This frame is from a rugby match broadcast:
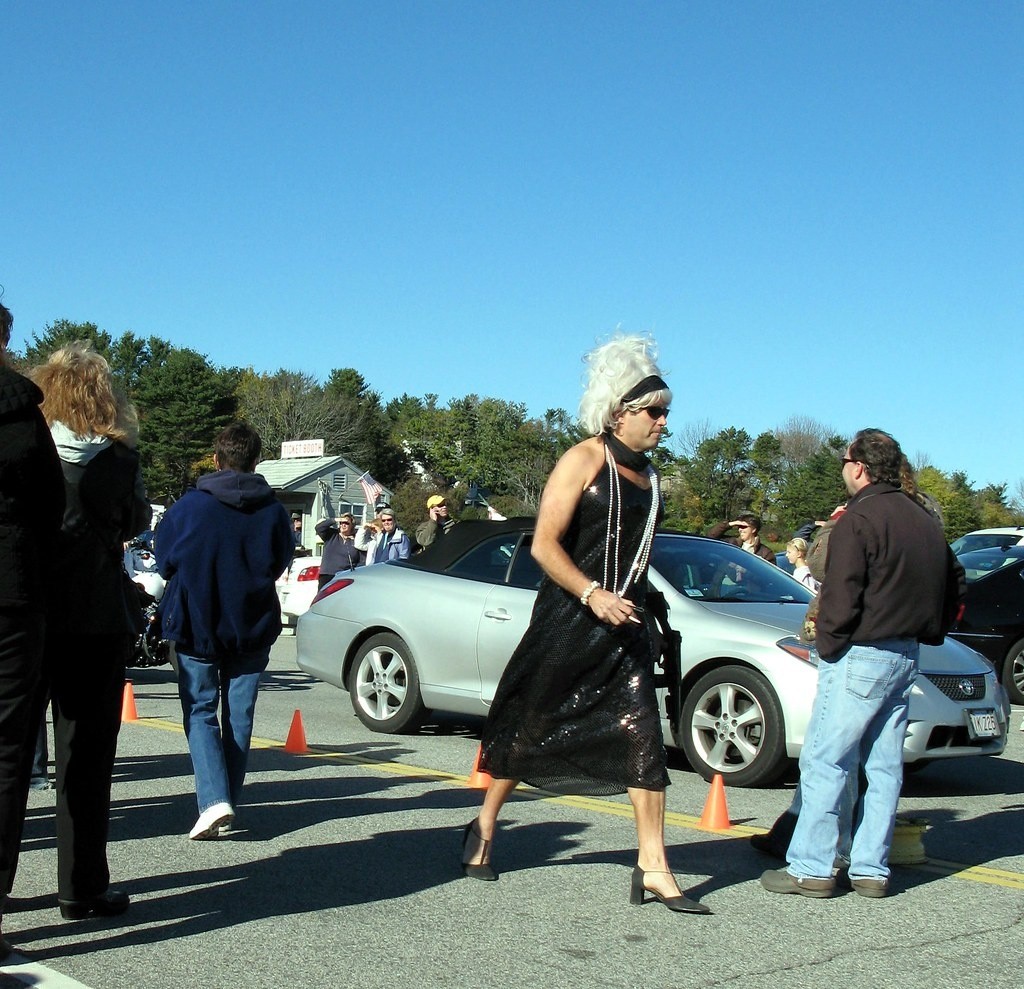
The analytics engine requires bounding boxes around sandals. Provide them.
[760,869,837,898]
[839,867,888,898]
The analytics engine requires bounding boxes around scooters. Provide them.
[129,529,167,665]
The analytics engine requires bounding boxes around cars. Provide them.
[295,516,1012,788]
[278,556,323,618]
[947,527,1024,706]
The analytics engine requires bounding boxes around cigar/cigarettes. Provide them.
[628,616,641,624]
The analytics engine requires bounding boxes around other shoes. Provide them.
[219,824,232,832]
[29,779,55,790]
[189,802,235,840]
[751,834,785,860]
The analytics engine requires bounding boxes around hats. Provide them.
[374,502,391,513]
[427,495,447,508]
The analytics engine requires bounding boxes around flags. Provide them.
[360,473,382,504]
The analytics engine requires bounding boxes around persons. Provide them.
[0,303,68,963]
[354,502,411,566]
[416,495,460,552]
[751,428,965,898]
[154,423,296,839]
[708,514,776,598]
[784,537,821,592]
[6,347,153,920]
[290,510,301,545]
[315,513,360,592]
[460,338,710,912]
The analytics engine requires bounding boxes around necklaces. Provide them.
[603,444,659,598]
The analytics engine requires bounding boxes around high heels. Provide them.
[629,865,710,913]
[58,891,129,918]
[462,818,498,881]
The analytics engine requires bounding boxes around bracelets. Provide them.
[581,581,601,606]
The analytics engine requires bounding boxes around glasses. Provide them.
[382,519,393,522]
[631,406,669,420]
[842,457,869,469]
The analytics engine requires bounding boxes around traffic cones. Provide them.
[121,682,138,721]
[283,709,311,754]
[700,774,732,828]
